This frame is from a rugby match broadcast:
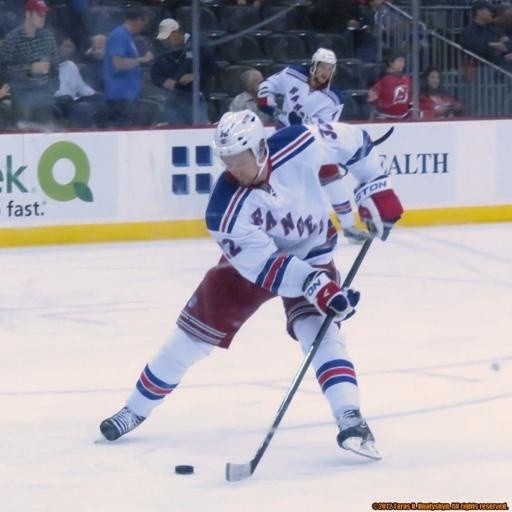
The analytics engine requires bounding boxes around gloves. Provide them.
[353,174,404,242]
[302,270,360,323]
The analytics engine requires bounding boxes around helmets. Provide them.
[310,47,337,75]
[213,110,266,158]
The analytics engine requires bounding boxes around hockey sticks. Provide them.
[226,230,375,480]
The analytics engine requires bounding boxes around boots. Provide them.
[100,405,150,441]
[335,406,375,449]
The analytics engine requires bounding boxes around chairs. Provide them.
[0,4,386,128]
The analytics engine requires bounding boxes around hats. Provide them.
[24,0,49,13]
[472,0,498,12]
[156,18,180,40]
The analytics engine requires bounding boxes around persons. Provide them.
[100,109,403,449]
[1,0,512,135]
[256,48,369,242]
